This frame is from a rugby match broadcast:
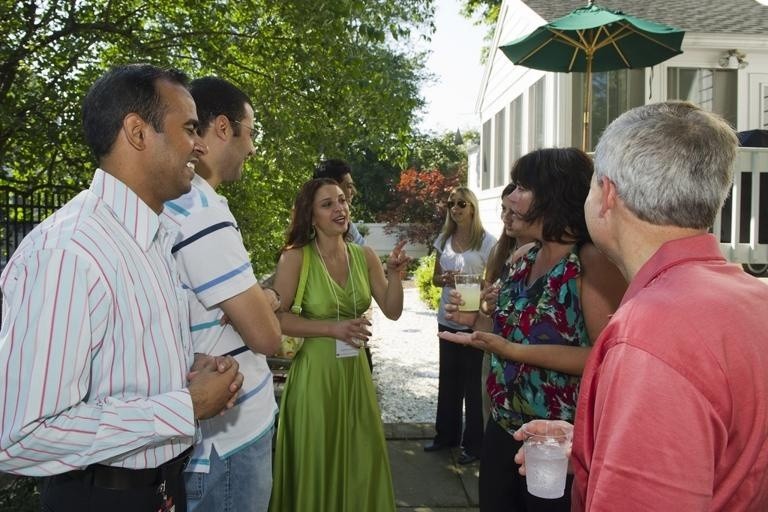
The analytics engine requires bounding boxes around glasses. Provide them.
[447,200,470,208]
[216,113,260,143]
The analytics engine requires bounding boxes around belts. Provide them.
[84,444,195,491]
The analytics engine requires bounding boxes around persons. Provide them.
[269,176,414,511]
[159,74,283,510]
[2,62,245,510]
[424,185,500,468]
[479,182,544,436]
[434,146,631,512]
[511,99,768,511]
[305,156,379,372]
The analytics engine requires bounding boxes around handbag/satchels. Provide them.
[266,245,311,360]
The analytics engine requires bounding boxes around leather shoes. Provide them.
[424,441,441,451]
[457,450,477,465]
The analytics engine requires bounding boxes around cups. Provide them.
[354,314,376,347]
[522,420,573,498]
[454,274,481,311]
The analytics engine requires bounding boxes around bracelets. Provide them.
[476,299,492,320]
[258,283,283,315]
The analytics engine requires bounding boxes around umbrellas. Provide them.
[497,1,688,153]
